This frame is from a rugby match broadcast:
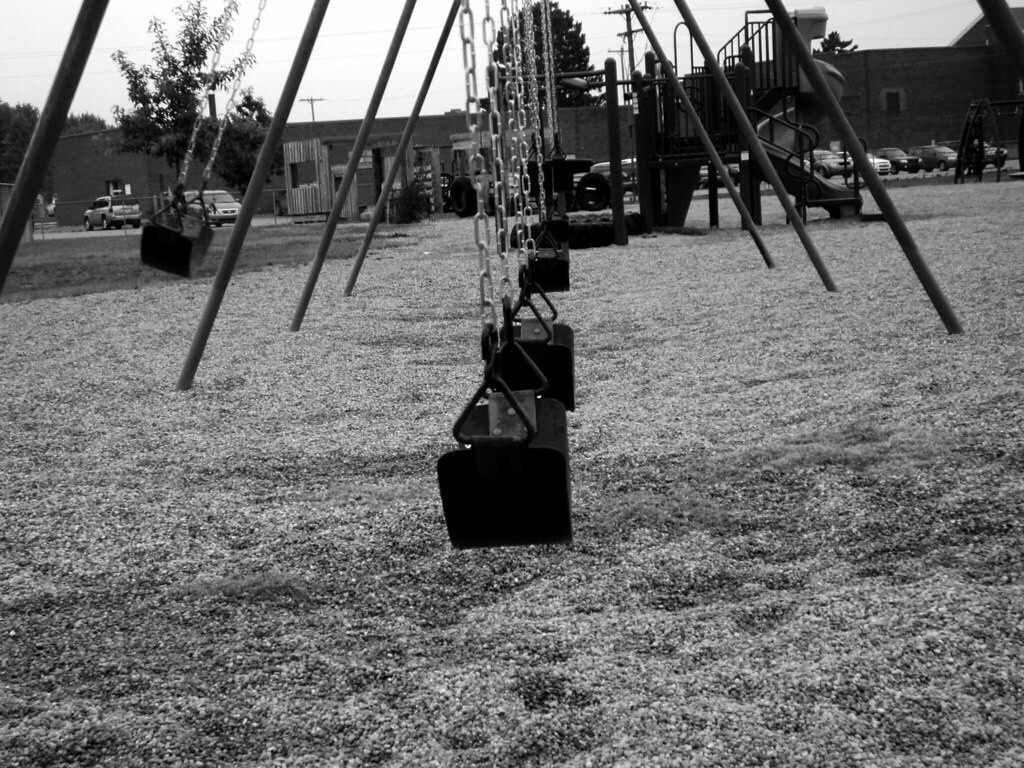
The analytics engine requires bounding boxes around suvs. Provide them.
[872,148,925,175]
[937,138,1008,168]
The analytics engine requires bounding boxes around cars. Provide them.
[82,194,141,230]
[804,150,856,180]
[837,151,892,175]
[180,189,242,228]
[566,154,744,199]
[46,196,56,216]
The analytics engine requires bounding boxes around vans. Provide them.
[907,145,959,172]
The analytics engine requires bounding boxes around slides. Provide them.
[748,55,863,213]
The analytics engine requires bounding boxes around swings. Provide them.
[431,0,580,554]
[136,0,268,280]
